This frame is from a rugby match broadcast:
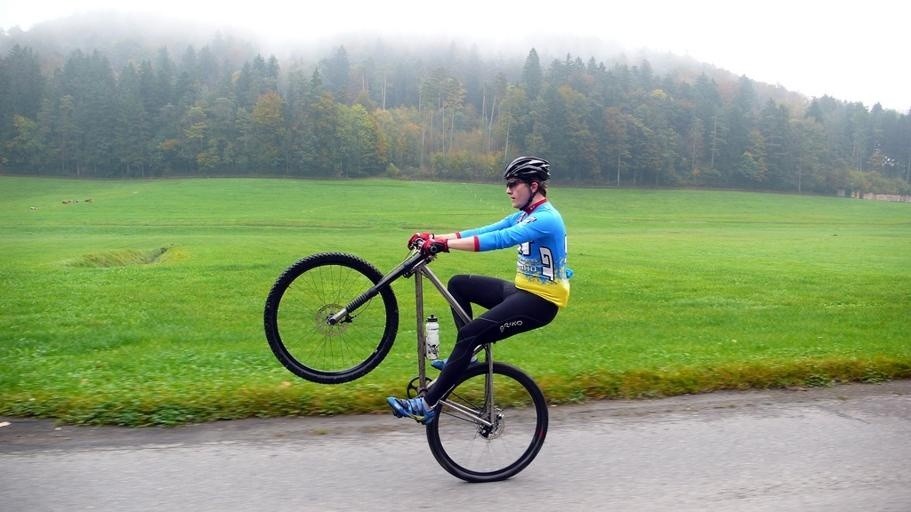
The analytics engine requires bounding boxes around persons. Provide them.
[385,154,574,427]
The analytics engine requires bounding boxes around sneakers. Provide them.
[386,393,438,427]
[431,354,480,372]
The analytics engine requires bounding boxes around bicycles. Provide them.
[262,231,550,484]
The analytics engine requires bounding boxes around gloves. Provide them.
[418,238,452,256]
[406,230,429,251]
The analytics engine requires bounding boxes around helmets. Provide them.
[503,154,552,184]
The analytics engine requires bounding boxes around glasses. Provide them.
[505,178,527,189]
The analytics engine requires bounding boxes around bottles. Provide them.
[425,314,441,360]
